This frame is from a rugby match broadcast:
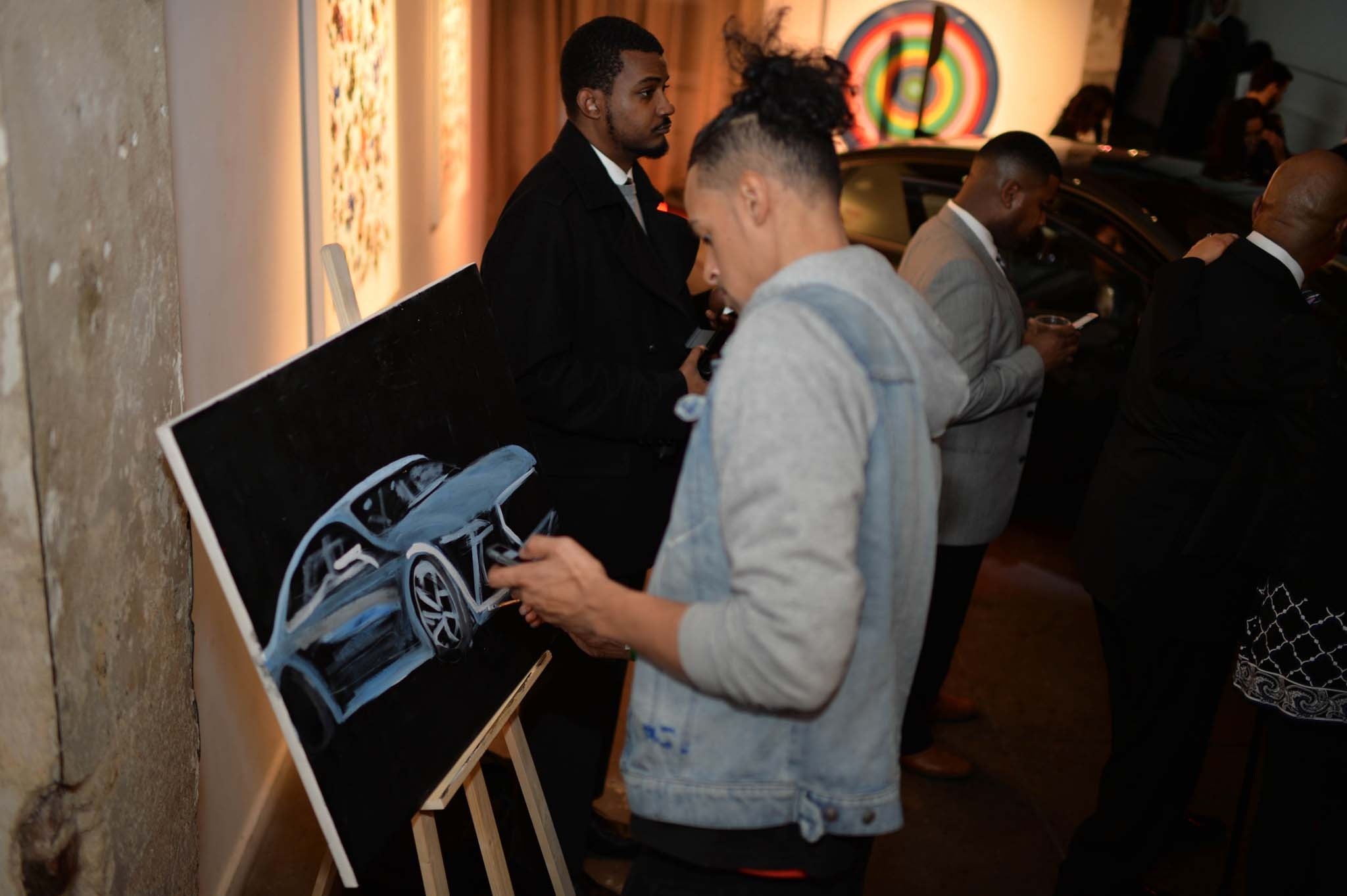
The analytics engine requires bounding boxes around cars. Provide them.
[262,444,562,760]
[837,132,1253,533]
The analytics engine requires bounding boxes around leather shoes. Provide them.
[931,690,979,719]
[900,744,973,780]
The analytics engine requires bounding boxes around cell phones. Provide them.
[1071,312,1099,330]
[485,542,536,567]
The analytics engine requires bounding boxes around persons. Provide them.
[901,131,1076,780]
[482,16,1347,896]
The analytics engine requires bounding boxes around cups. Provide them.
[1034,314,1072,339]
